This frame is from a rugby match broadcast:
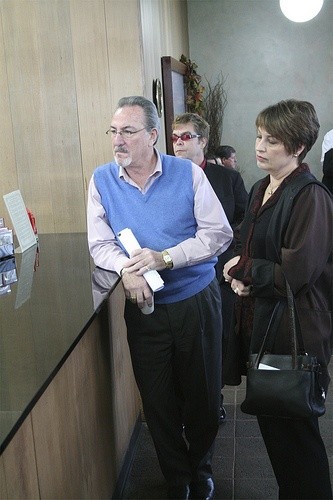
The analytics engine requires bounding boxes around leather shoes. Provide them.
[165,477,217,500]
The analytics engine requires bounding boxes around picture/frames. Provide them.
[162,55,188,156]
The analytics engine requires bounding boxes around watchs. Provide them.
[161,250,172,269]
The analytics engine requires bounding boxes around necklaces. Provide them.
[268,184,279,194]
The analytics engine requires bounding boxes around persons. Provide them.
[320,129,333,194]
[167,112,249,421]
[222,100,333,500]
[87,95,233,500]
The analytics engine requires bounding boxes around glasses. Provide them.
[169,131,200,142]
[105,126,147,137]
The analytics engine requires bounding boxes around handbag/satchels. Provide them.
[240,276,331,420]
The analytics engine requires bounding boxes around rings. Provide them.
[144,266,149,271]
[130,297,137,300]
[225,279,228,282]
[234,288,238,294]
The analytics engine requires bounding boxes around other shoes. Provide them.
[219,406,227,422]
